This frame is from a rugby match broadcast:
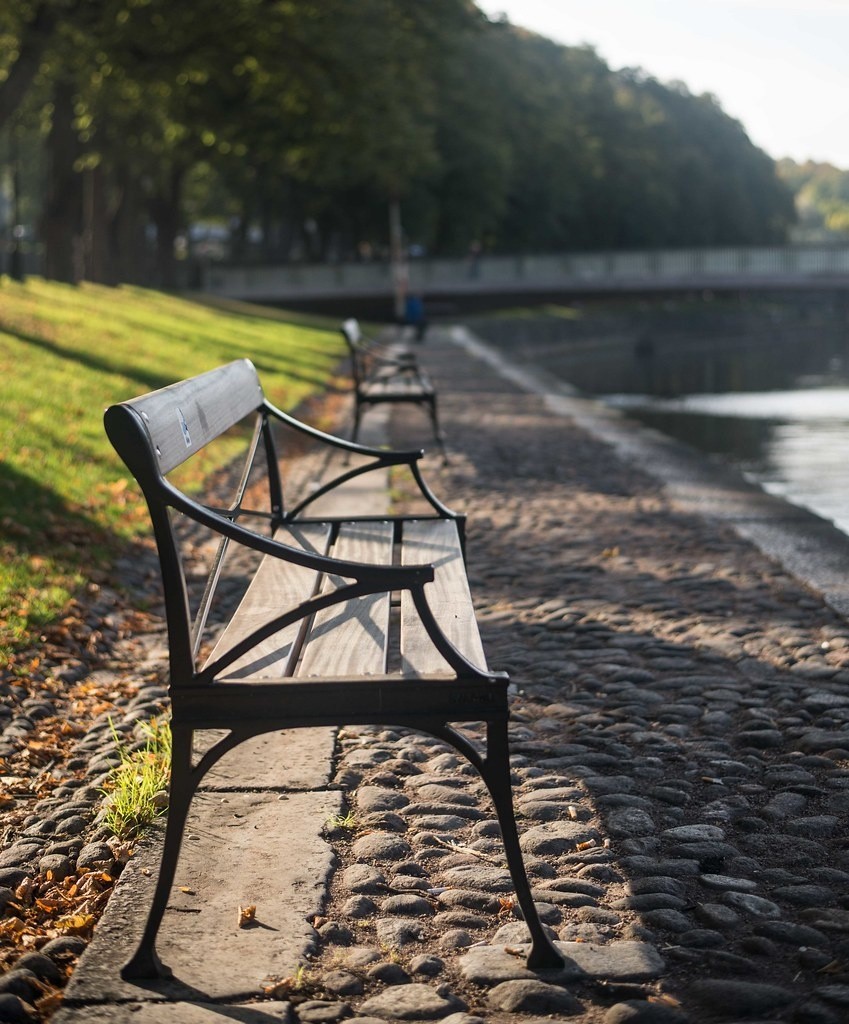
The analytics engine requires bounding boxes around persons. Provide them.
[405,292,426,342]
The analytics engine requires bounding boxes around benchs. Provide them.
[340,318,450,467]
[101,353,568,980]
